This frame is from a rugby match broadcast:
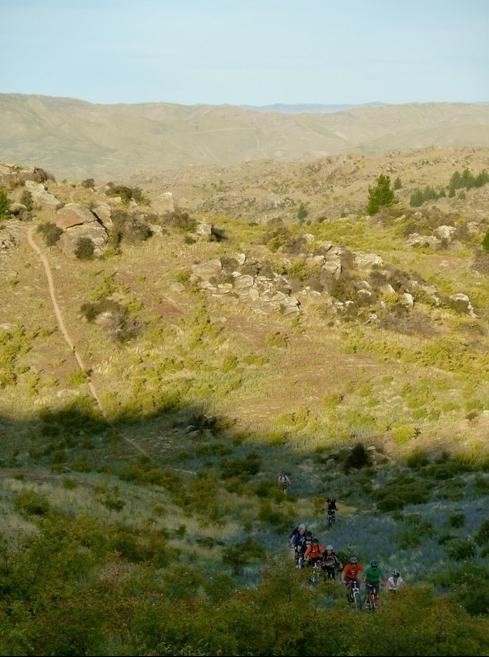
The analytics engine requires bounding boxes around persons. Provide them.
[290,522,406,611]
[326,491,339,528]
[279,470,291,494]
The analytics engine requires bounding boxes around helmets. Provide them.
[298,523,320,544]
[391,570,400,577]
[350,557,358,563]
[325,545,334,550]
[371,560,378,566]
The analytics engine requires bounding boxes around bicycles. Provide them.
[328,509,336,530]
[289,543,341,599]
[365,582,379,611]
[347,578,361,610]
